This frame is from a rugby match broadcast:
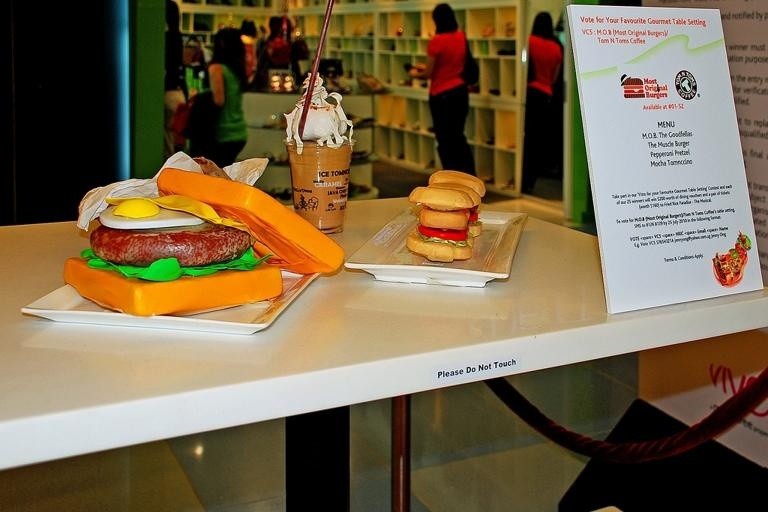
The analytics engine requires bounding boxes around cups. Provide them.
[281,137,358,236]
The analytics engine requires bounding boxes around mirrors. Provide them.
[525,0,570,197]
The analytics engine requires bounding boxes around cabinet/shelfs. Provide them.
[237,96,376,199]
[375,2,527,197]
[286,0,374,93]
[175,0,285,96]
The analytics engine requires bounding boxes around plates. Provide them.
[341,203,530,291]
[17,265,321,336]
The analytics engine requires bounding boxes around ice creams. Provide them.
[287,74,349,235]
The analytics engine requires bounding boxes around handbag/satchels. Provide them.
[465,39,480,86]
[268,16,310,65]
[173,93,199,143]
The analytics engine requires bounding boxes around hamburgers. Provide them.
[620,74,646,99]
[62,156,345,316]
[406,168,488,262]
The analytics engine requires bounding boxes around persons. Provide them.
[191,25,249,171]
[238,17,266,85]
[407,2,479,180]
[289,17,311,88]
[164,0,190,158]
[519,11,564,195]
[252,15,293,91]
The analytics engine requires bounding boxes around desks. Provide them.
[0,198,768,512]
